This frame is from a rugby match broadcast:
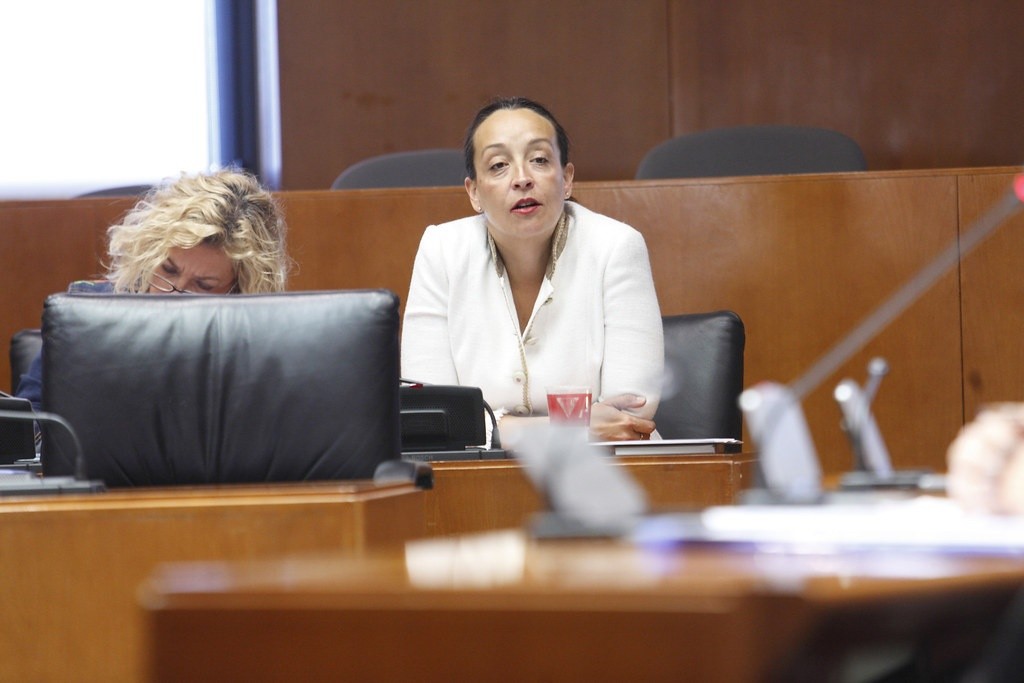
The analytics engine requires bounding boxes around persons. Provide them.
[879,401,1024,683]
[19,171,295,452]
[399,97,665,440]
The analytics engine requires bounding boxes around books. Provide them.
[614,445,726,454]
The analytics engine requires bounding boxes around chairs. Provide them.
[11,292,398,488]
[634,125,865,183]
[329,147,465,190]
[655,312,745,453]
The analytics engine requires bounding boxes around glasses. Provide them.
[140,268,238,296]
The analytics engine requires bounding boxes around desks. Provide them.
[0,486,427,683]
[129,503,1024,683]
[428,458,758,542]
[0,165,1024,471]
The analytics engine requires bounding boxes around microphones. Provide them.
[740,174,1024,507]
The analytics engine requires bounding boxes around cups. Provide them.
[546,386,592,450]
